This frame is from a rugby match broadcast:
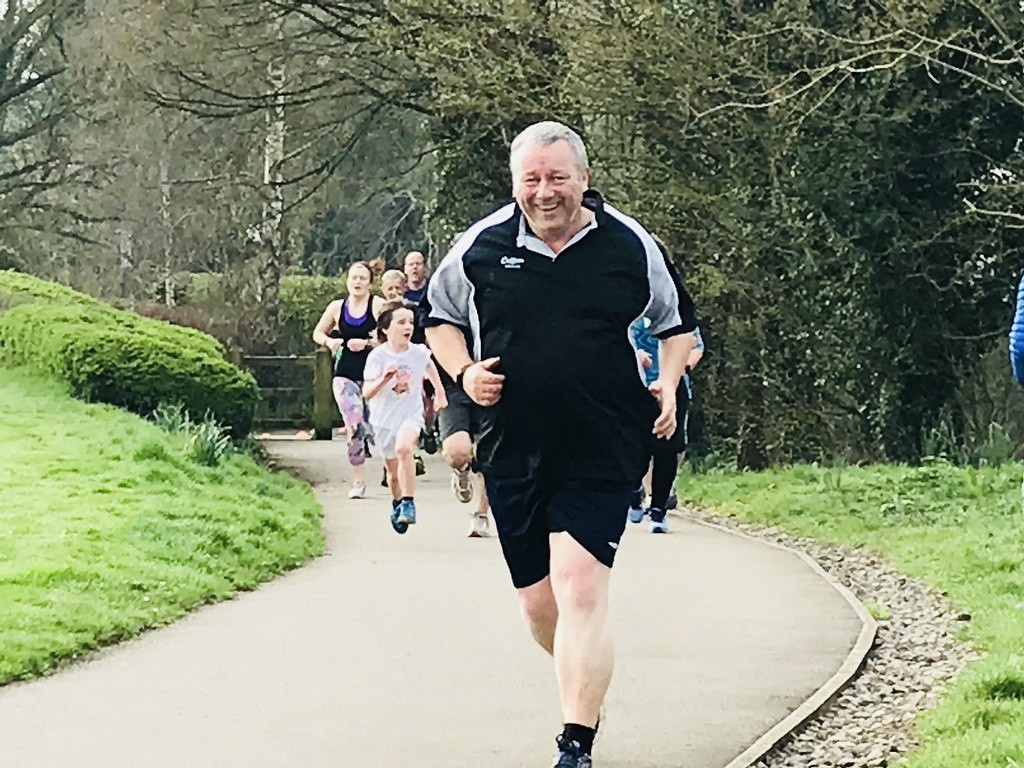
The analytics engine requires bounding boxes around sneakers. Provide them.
[468,511,488,537]
[391,500,408,533]
[451,467,472,503]
[628,485,646,522]
[650,508,667,532]
[348,482,367,499]
[397,500,415,524]
[382,467,388,488]
[552,733,593,768]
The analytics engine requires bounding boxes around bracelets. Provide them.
[323,337,331,346]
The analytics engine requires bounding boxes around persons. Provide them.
[314,232,703,537]
[1009,274,1024,385]
[420,119,698,768]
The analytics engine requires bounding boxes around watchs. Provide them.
[456,362,473,392]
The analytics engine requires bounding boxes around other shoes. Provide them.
[414,455,424,475]
[421,428,438,454]
[666,495,678,509]
[363,441,371,457]
